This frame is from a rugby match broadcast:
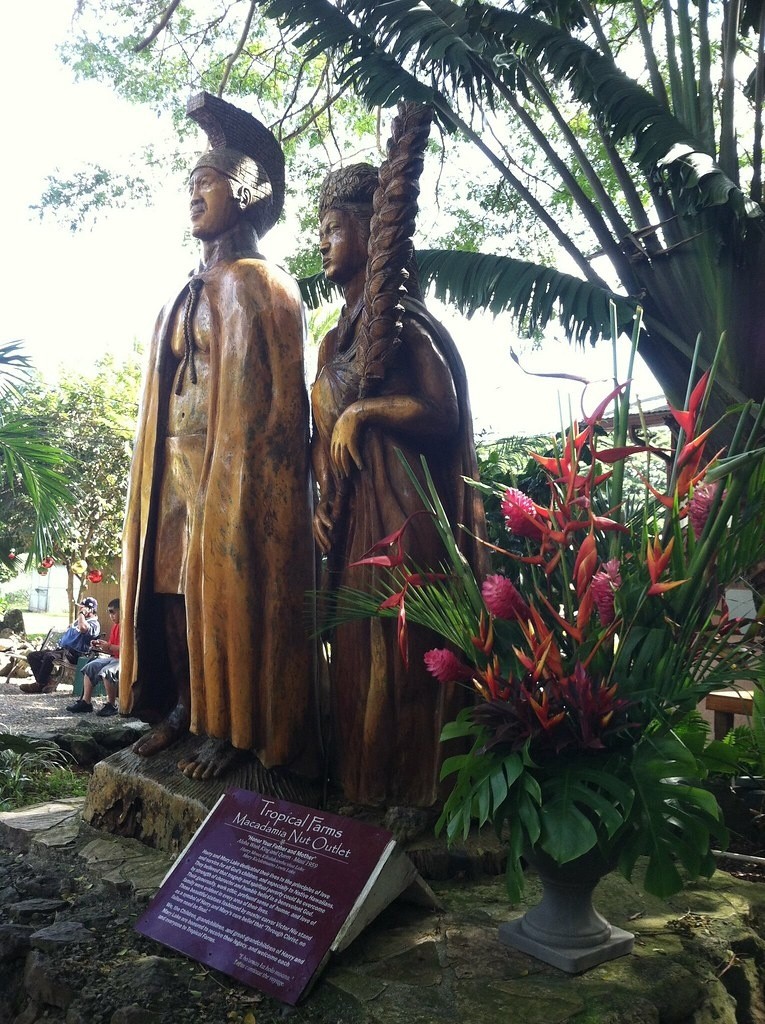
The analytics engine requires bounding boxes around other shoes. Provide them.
[42,680,56,693]
[20,682,41,693]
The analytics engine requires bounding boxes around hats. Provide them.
[74,597,97,611]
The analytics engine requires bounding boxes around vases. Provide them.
[497,797,633,974]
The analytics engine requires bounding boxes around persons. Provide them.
[19,596,100,694]
[64,598,121,716]
[116,92,320,778]
[308,163,488,846]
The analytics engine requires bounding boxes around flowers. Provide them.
[296,296,765,909]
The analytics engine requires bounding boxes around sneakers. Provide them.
[67,700,93,713]
[96,702,118,716]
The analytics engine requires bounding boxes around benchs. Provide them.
[5,625,106,684]
